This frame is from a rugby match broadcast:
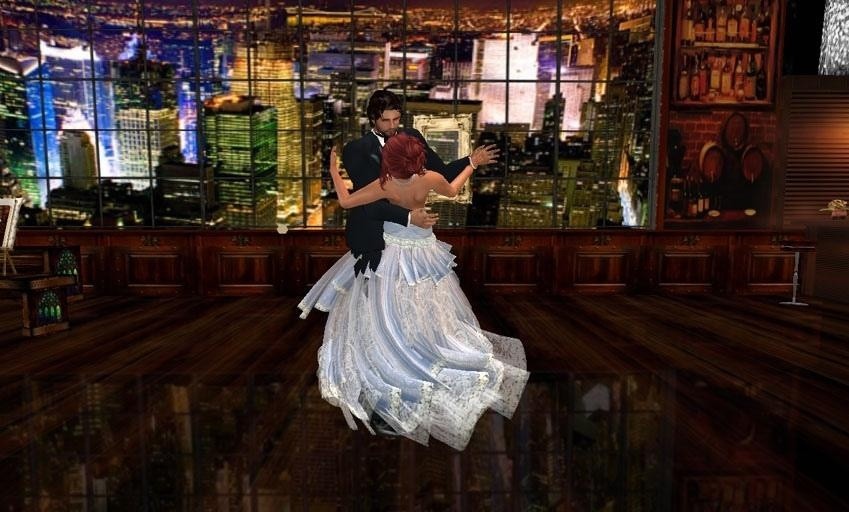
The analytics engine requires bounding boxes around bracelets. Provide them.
[467,156,478,171]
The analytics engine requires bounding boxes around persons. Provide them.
[340,87,503,290]
[329,141,501,392]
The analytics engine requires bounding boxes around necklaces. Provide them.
[390,174,418,186]
